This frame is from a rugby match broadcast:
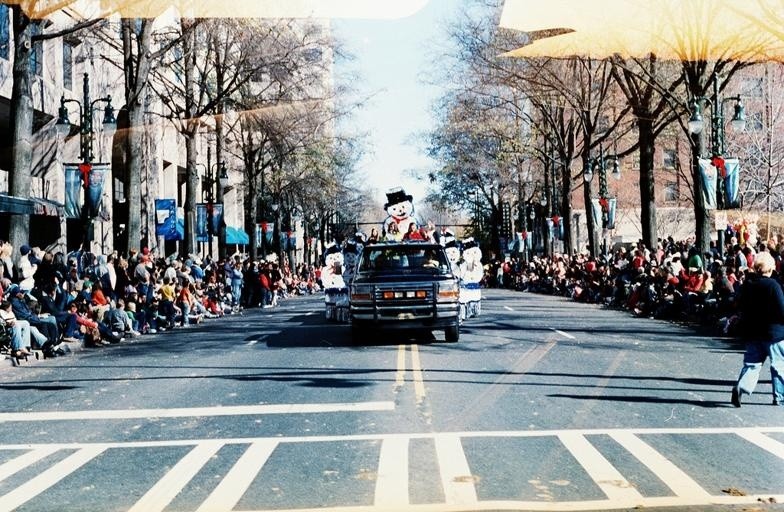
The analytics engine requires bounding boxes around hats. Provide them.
[343,242,360,255]
[442,240,459,249]
[325,244,340,257]
[19,244,33,256]
[462,240,480,252]
[383,188,413,211]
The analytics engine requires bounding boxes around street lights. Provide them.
[582,139,622,261]
[687,71,748,253]
[56,71,121,251]
[196,146,229,256]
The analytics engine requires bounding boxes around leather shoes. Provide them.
[772,400,781,406]
[730,386,742,408]
[13,349,34,360]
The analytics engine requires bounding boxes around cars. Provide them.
[348,241,461,343]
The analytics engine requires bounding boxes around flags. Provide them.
[514,157,741,256]
[61,160,297,250]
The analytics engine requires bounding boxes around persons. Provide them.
[482,222,783,354]
[1,234,326,354]
[364,221,444,269]
[730,250,784,408]
